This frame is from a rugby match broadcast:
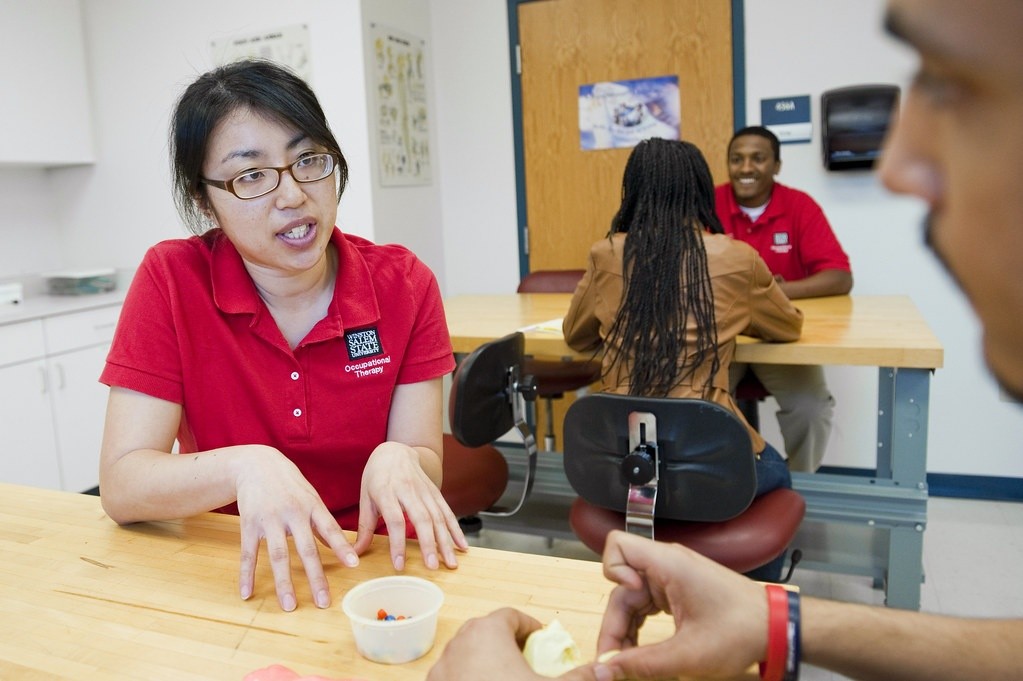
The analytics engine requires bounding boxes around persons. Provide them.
[427,0,1023,681]
[715,125,853,473]
[561,138,803,583]
[97,62,468,612]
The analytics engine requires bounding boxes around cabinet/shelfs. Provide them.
[0,0,94,170]
[0,304,124,499]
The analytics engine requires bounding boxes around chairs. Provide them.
[515,271,588,457]
[563,394,805,584]
[440,331,536,531]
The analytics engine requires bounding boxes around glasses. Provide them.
[199,151,338,200]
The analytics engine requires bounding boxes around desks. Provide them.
[440,292,943,612]
[0,481,758,680]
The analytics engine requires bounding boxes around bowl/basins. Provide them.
[342,576,444,662]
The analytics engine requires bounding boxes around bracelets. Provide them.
[759,586,801,681]
